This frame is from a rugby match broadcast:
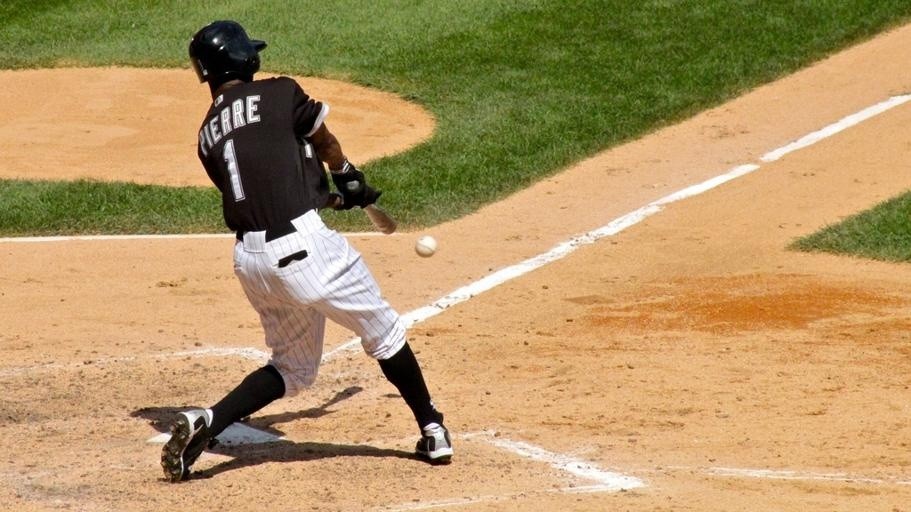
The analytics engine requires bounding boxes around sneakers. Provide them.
[415,429,454,462]
[161,410,209,481]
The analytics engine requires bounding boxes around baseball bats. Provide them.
[363,204,397,234]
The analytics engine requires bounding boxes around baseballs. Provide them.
[415,236,436,257]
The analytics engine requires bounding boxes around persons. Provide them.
[160,20,454,481]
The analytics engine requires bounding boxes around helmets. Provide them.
[188,19,267,82]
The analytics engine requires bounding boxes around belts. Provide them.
[236,218,298,244]
